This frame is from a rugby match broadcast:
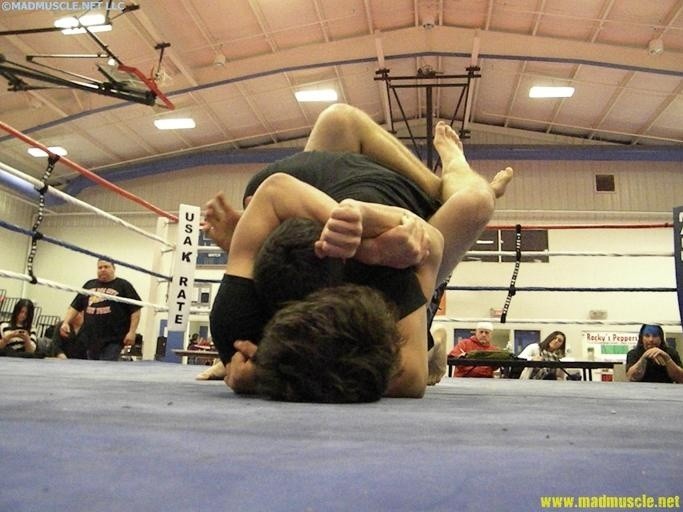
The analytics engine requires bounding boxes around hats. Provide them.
[477,322,493,331]
[642,324,662,336]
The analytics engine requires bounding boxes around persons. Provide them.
[0,298,37,356]
[187,333,204,351]
[625,324,683,385]
[444,319,509,378]
[206,98,512,403]
[52,310,83,360]
[59,257,142,361]
[197,147,448,389]
[515,329,568,381]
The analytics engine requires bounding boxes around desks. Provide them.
[169,345,622,381]
